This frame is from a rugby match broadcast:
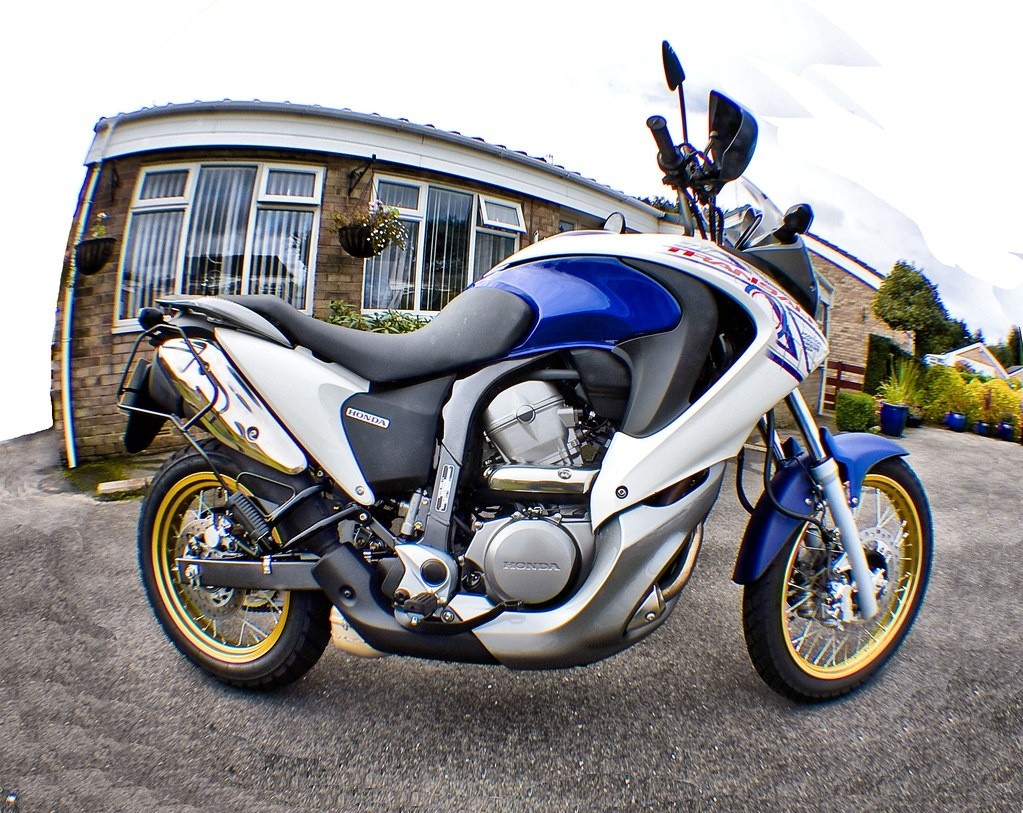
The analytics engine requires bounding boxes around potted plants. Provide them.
[907,407,923,427]
[878,360,919,437]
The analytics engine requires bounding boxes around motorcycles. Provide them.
[116,38,935,707]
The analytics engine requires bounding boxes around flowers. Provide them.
[324,197,415,257]
[91,211,108,238]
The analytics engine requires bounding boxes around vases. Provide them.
[337,224,389,257]
[946,412,967,432]
[72,236,117,276]
[977,423,992,436]
[997,422,1014,442]
